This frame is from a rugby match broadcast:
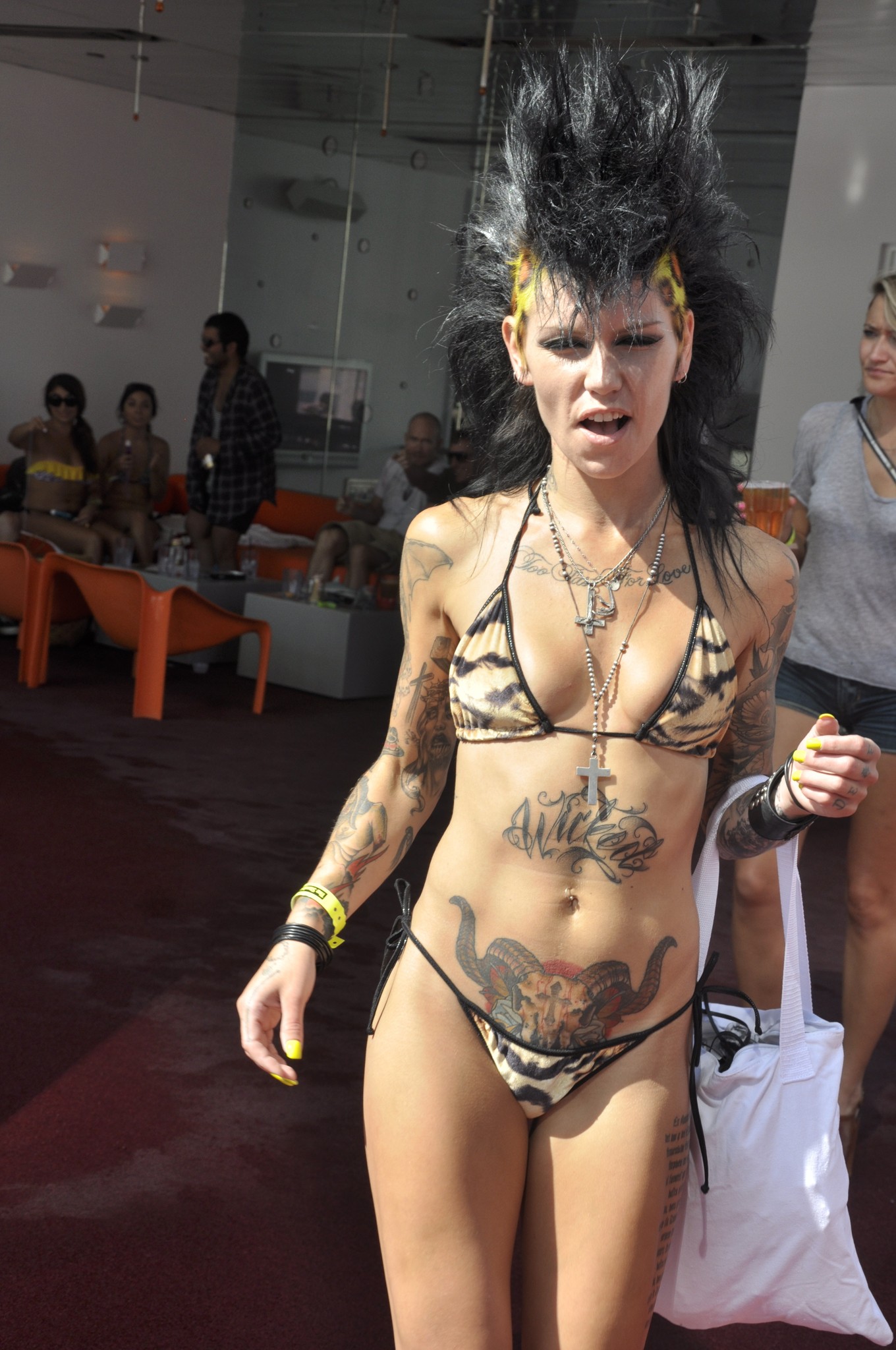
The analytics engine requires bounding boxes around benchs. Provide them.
[151,473,400,610]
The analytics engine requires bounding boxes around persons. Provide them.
[308,412,479,591]
[735,274,896,1173]
[235,50,881,1349]
[184,310,282,572]
[0,372,171,567]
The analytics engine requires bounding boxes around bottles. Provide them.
[166,538,186,579]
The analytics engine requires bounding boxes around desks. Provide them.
[98,557,285,675]
[236,591,406,701]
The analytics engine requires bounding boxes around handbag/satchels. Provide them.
[641,768,893,1344]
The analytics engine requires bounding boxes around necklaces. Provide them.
[541,464,672,807]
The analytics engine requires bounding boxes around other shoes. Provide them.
[839,1105,860,1175]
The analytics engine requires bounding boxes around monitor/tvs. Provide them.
[257,353,375,468]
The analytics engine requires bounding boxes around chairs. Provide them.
[0,539,270,722]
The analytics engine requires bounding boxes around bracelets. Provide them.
[784,747,807,811]
[290,883,347,948]
[746,764,819,841]
[267,924,334,976]
[784,525,796,546]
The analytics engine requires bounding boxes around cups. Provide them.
[741,481,791,539]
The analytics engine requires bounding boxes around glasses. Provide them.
[47,395,78,407]
[204,339,225,348]
[448,451,470,462]
